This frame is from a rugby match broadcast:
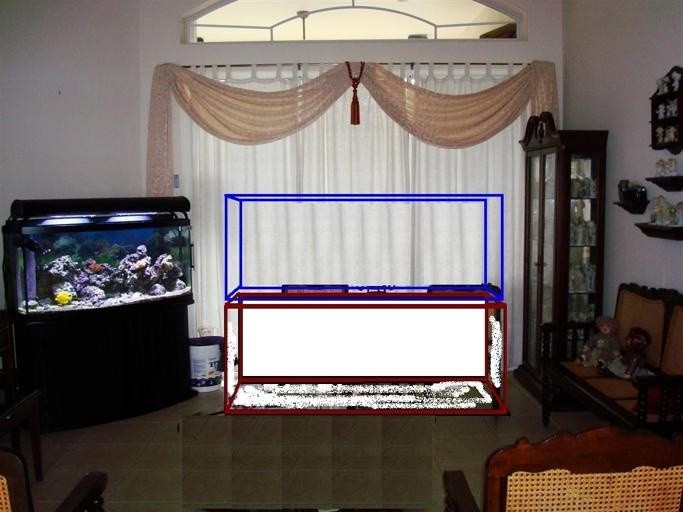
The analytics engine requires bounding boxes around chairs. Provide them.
[442,426,683,512]
[0,447,108,512]
[0,309,44,482]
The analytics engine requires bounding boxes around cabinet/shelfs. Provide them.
[514,109,608,428]
[613,174,683,241]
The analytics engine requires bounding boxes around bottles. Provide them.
[569,246,595,292]
[571,200,596,246]
[569,294,595,321]
[570,156,597,198]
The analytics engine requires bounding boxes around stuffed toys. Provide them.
[599,326,656,379]
[578,316,621,370]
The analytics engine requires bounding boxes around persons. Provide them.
[647,70,682,230]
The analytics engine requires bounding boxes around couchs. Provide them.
[540,282,683,441]
